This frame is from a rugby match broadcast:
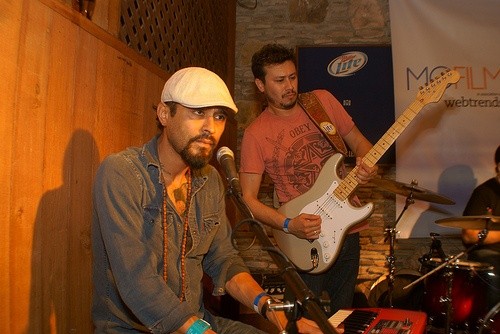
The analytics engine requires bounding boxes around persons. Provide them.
[461,147,500,306]
[238,43,379,323]
[91,68,344,334]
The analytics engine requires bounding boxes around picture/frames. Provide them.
[295,44,396,167]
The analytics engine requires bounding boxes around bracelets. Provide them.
[253,292,271,318]
[261,298,275,320]
[283,219,291,234]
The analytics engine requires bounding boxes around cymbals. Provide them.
[366,178,457,205]
[435,216,500,232]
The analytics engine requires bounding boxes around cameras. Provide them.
[216,145,241,192]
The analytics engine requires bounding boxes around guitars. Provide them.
[272,69,460,275]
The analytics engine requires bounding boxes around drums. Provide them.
[479,302,500,334]
[422,258,495,329]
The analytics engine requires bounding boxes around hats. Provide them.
[161,67,238,114]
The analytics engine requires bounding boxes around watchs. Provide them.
[186,318,213,334]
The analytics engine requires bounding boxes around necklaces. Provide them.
[159,169,191,306]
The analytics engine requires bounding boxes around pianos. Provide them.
[328,308,428,334]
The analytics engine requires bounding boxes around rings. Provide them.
[314,231,317,235]
